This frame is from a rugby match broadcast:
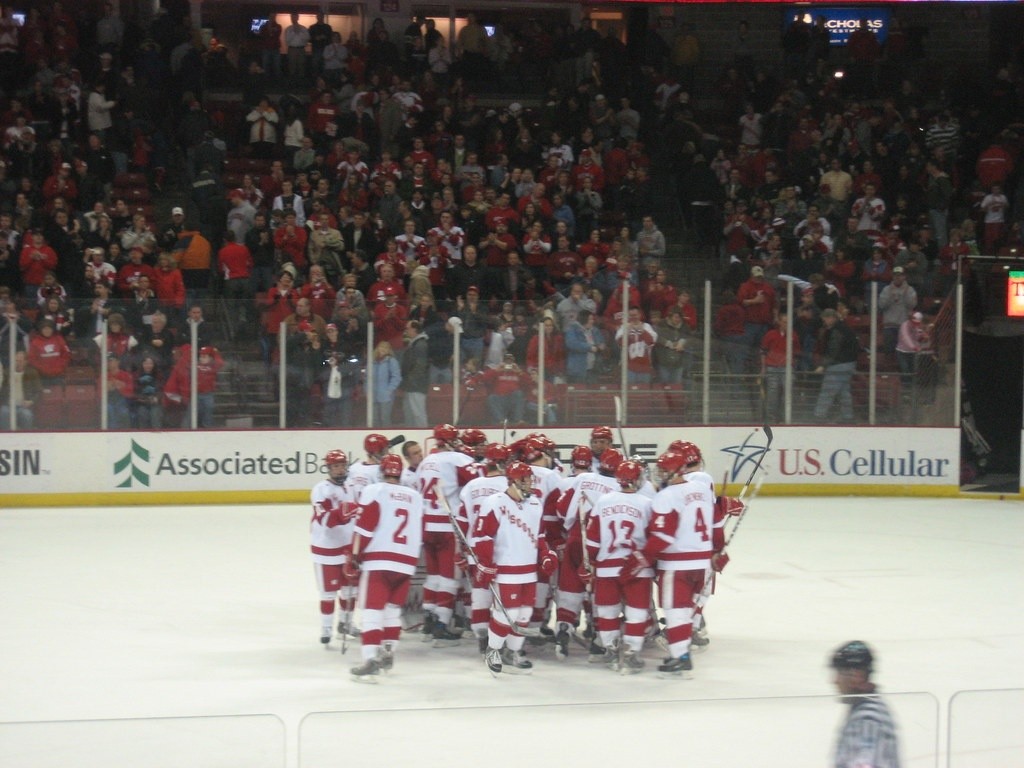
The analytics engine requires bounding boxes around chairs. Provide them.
[0,93,1024,423]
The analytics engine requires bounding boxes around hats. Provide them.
[449,316,464,334]
[172,208,184,216]
[830,641,873,673]
[751,265,765,277]
[892,265,905,274]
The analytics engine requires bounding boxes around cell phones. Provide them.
[504,365,513,370]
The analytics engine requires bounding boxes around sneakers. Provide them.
[337,623,361,640]
[525,627,711,678]
[320,627,334,650]
[350,650,396,683]
[422,615,488,652]
[484,648,533,679]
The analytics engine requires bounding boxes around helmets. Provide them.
[573,424,702,491]
[365,434,389,455]
[325,450,347,465]
[434,423,557,485]
[379,454,403,477]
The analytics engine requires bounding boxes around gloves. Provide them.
[474,562,497,590]
[618,550,650,585]
[327,509,350,527]
[711,550,729,573]
[718,496,743,517]
[343,562,360,582]
[577,560,598,584]
[538,549,559,575]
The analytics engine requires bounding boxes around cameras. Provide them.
[735,222,741,226]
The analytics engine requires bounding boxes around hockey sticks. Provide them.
[722,426,773,528]
[389,435,405,448]
[614,396,627,459]
[341,578,351,655]
[503,418,508,445]
[695,470,767,612]
[433,485,540,638]
[579,498,592,594]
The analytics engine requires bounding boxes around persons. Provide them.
[828,641,901,768]
[0,1,1024,486]
[310,422,743,676]
